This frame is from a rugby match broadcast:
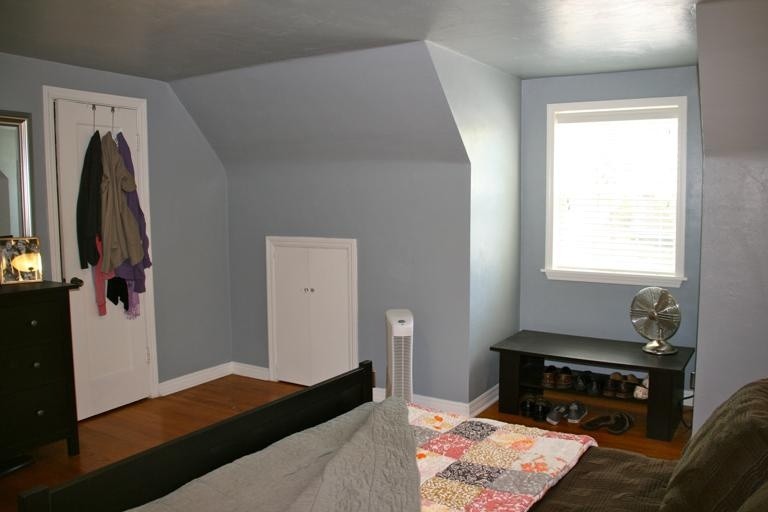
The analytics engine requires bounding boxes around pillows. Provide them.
[660,377,768,511]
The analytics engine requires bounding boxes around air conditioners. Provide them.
[385,310,414,402]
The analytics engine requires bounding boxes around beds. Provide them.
[0,358,767,511]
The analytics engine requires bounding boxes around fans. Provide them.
[629,286,682,355]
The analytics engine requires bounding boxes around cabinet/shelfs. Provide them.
[1,278,85,457]
[489,329,695,441]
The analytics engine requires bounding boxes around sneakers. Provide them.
[521,398,635,434]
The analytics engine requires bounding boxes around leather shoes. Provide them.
[542,365,649,400]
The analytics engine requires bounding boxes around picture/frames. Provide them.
[0,109,36,238]
